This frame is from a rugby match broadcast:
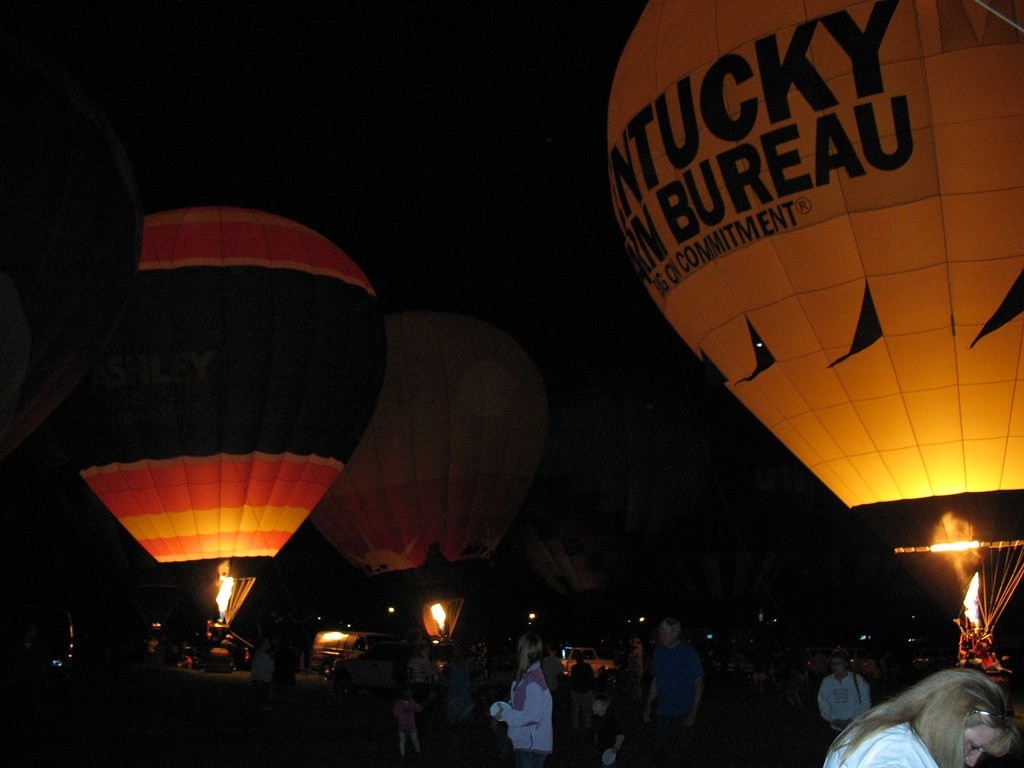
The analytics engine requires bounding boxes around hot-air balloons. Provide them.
[0,93,145,456]
[605,0,1024,695]
[34,203,389,647]
[306,308,550,663]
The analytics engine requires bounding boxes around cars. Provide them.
[309,630,402,678]
[556,647,617,679]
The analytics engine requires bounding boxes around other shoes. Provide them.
[572,724,591,728]
[402,752,422,758]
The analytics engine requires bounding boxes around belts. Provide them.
[577,689,589,694]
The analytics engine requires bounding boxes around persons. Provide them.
[146,629,1024,768]
[642,618,705,768]
